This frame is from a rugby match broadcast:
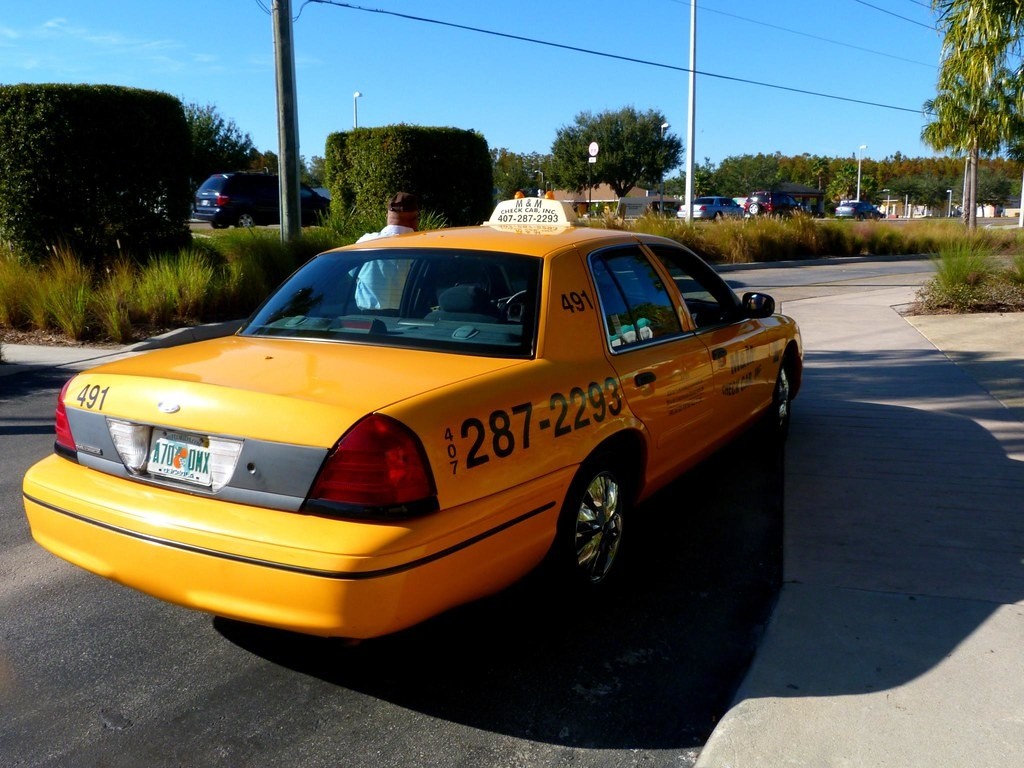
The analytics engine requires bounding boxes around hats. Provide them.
[387,192,417,213]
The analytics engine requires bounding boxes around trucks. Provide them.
[744,191,799,217]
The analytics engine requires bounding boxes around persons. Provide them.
[343,191,421,318]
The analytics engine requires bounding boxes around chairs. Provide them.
[424,287,494,322]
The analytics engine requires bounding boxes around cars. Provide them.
[22,196,803,647]
[835,202,882,222]
[677,196,743,221]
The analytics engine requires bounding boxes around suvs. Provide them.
[192,169,333,229]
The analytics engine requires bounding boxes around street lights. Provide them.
[534,170,545,189]
[353,90,363,129]
[947,189,952,218]
[961,157,971,223]
[856,144,868,203]
[883,188,891,220]
[660,122,671,210]
